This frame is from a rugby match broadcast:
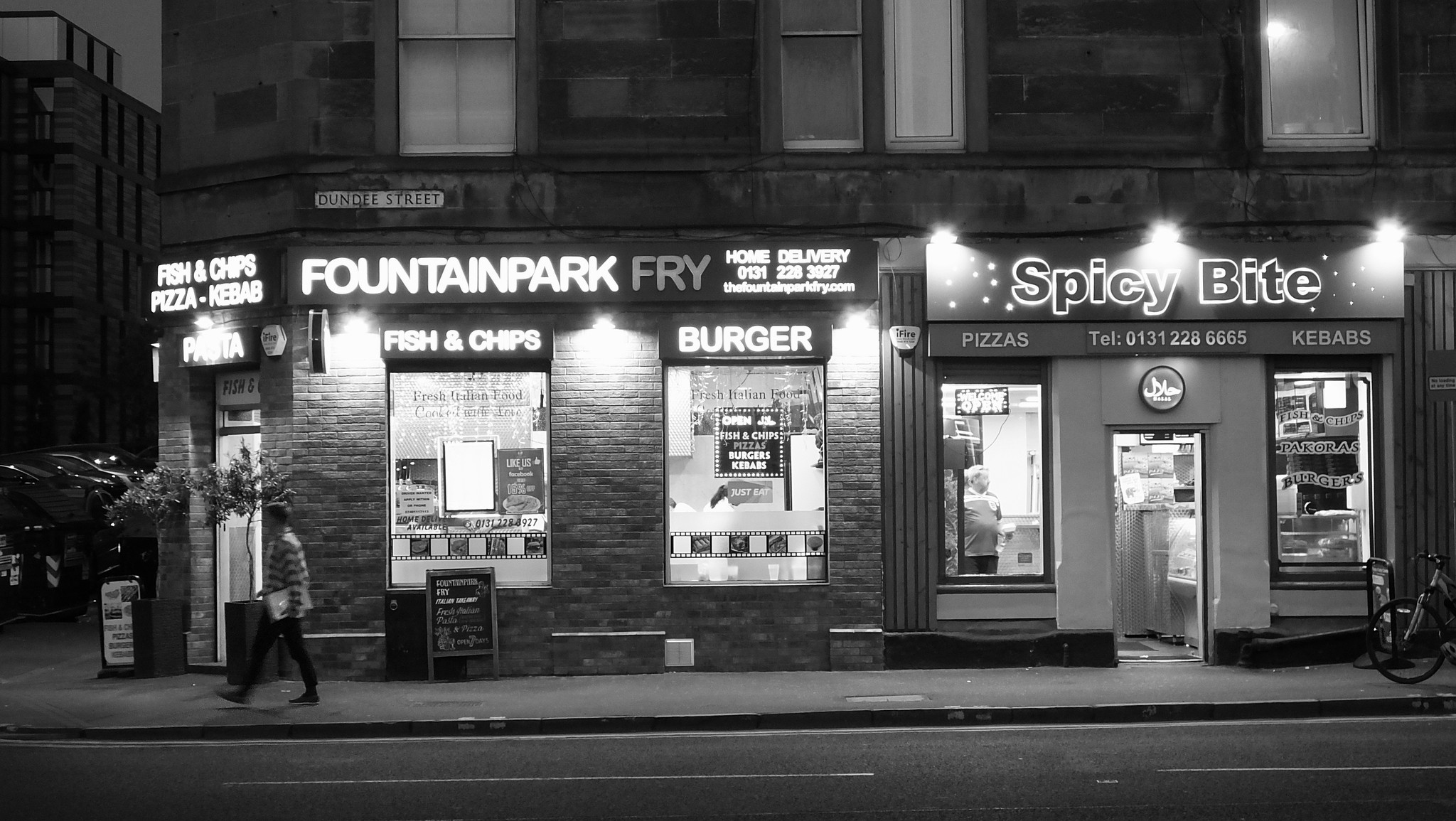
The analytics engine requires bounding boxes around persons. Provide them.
[217,502,320,703]
[965,465,1002,574]
[702,485,740,512]
[669,498,696,512]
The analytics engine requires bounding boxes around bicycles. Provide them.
[1366,552,1456,683]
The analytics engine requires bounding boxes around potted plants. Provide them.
[196,437,296,685]
[104,466,193,678]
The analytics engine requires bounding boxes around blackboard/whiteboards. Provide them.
[426,567,498,657]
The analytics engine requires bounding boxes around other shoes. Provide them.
[288,690,320,705]
[216,688,250,704]
